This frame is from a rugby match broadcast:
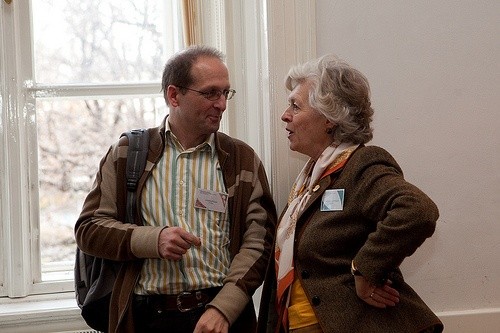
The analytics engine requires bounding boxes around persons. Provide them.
[74,46,278,333]
[255,54,444,333]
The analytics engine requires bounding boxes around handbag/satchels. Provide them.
[74,127,151,333]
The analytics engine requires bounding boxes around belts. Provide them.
[136,290,224,311]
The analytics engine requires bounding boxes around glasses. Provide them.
[174,85,236,102]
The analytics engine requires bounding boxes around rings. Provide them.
[370,293,373,298]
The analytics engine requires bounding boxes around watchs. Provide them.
[351,259,363,277]
